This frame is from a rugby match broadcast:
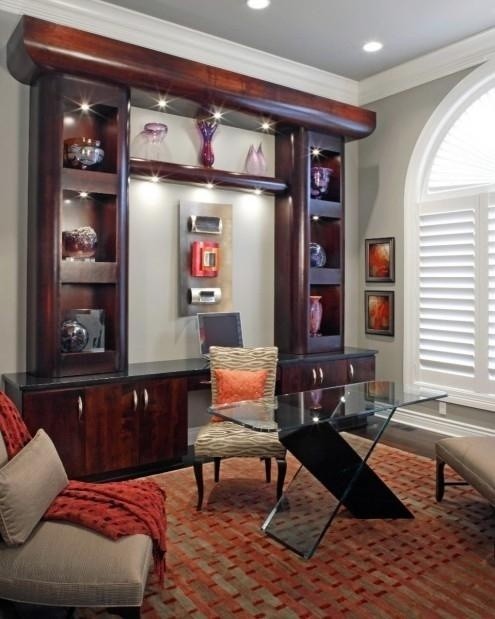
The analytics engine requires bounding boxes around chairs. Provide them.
[435,436,495,506]
[192,345,291,511]
[1,390,166,619]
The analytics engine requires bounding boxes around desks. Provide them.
[205,379,448,561]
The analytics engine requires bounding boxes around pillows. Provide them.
[209,368,270,423]
[2,427,68,549]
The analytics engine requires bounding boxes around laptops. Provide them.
[197,311,244,362]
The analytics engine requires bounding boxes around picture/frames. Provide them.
[366,237,394,283]
[364,290,394,336]
